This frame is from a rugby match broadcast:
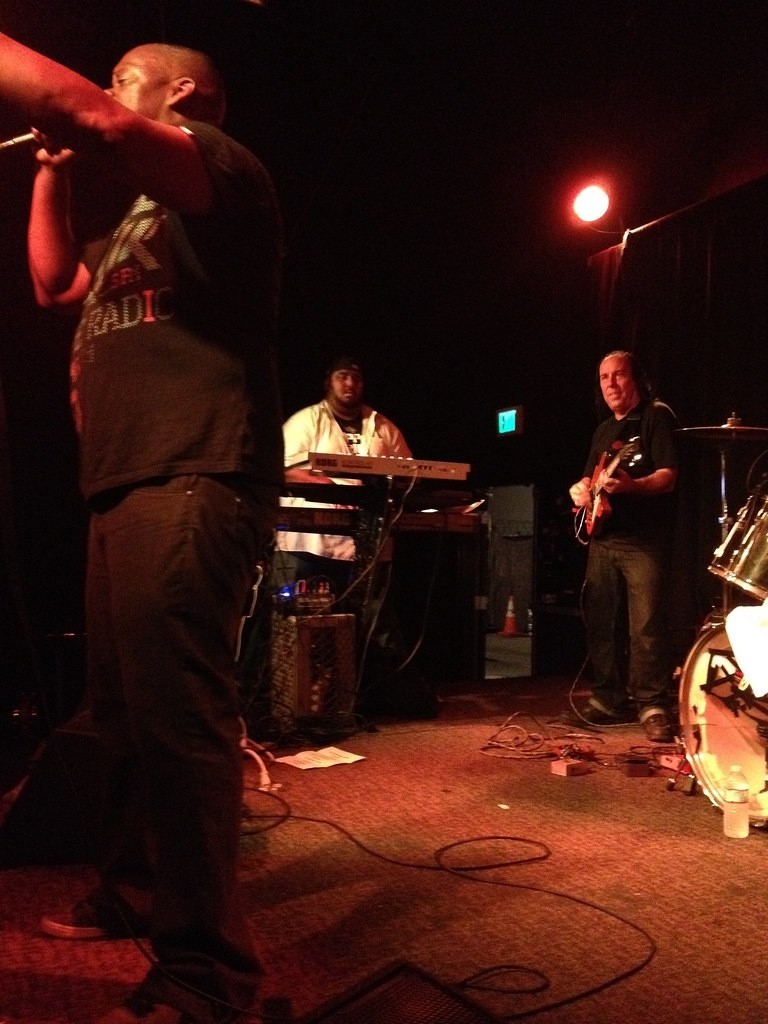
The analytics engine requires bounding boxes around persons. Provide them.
[281,356,415,743]
[0,29,287,1024]
[569,350,684,741]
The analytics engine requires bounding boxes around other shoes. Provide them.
[562,696,629,727]
[638,704,674,740]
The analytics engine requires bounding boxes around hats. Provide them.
[329,352,365,375]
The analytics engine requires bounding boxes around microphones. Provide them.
[0,133,42,157]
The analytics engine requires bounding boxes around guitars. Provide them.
[580,435,642,538]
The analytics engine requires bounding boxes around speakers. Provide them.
[291,959,501,1024]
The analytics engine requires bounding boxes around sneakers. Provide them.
[107,959,260,1022]
[41,885,155,941]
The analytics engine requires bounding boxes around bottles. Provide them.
[723,764,749,839]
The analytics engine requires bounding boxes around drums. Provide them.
[708,488,768,601]
[672,612,768,828]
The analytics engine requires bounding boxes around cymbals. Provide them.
[676,411,768,442]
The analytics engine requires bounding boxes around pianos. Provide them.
[273,479,494,536]
